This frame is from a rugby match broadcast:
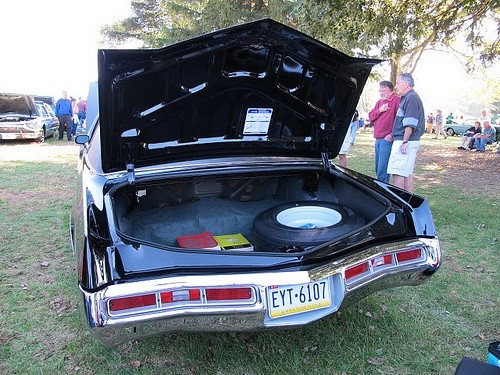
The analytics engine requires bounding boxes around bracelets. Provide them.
[402,141,407,144]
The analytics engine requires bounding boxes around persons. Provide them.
[339,124,351,168]
[55,91,74,141]
[475,121,496,153]
[432,109,447,139]
[387,73,425,192]
[67,96,87,137]
[358,116,375,133]
[350,109,359,146]
[368,81,400,184]
[479,111,491,130]
[457,121,482,151]
[424,112,464,135]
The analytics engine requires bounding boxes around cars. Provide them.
[443,118,482,136]
[0,93,60,143]
[67,17,442,350]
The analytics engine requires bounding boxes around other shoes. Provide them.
[67,138,75,142]
[457,146,466,150]
